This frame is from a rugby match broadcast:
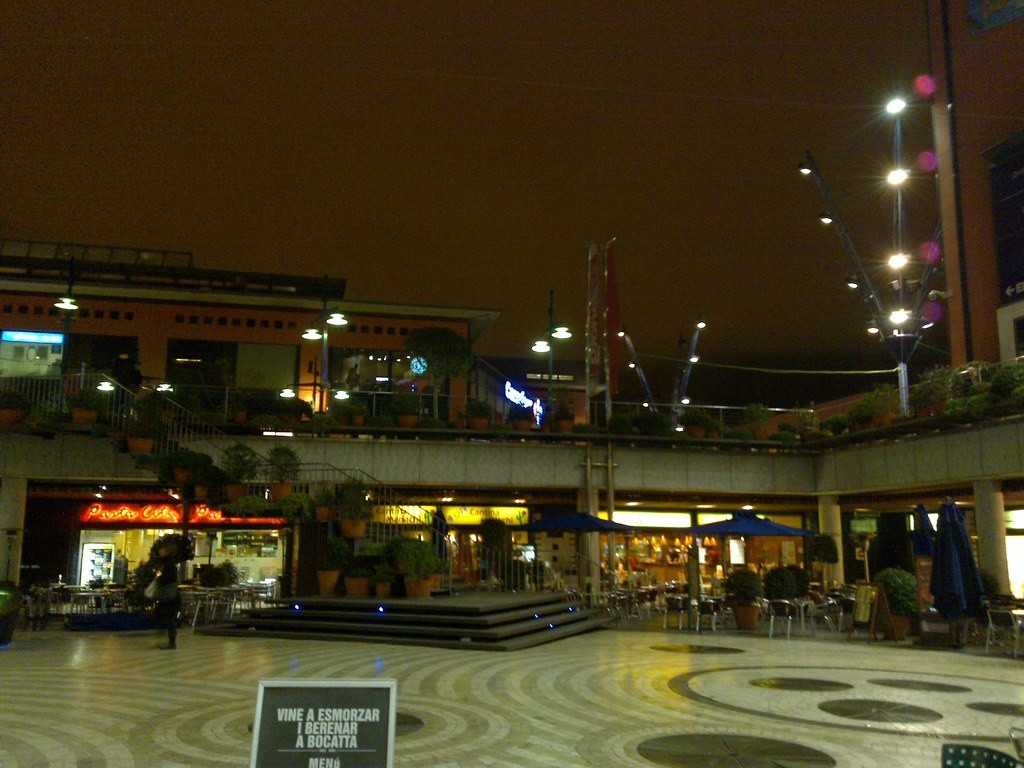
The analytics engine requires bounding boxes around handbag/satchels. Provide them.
[144,579,158,599]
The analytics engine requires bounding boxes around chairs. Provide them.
[600,579,856,639]
[984,609,1024,660]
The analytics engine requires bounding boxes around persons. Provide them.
[152,558,182,649]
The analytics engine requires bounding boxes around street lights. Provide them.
[531,289,573,421]
[53,256,81,410]
[302,296,349,416]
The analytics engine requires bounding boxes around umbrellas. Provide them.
[684,512,810,537]
[929,496,988,643]
[431,509,449,556]
[910,503,935,557]
[512,511,630,532]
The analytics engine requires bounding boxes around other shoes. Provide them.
[159,642,176,649]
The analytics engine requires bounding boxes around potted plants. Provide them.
[761,564,809,617]
[384,536,445,598]
[315,534,349,596]
[873,568,918,641]
[0,378,947,537]
[343,557,372,596]
[372,562,402,598]
[724,569,764,631]
